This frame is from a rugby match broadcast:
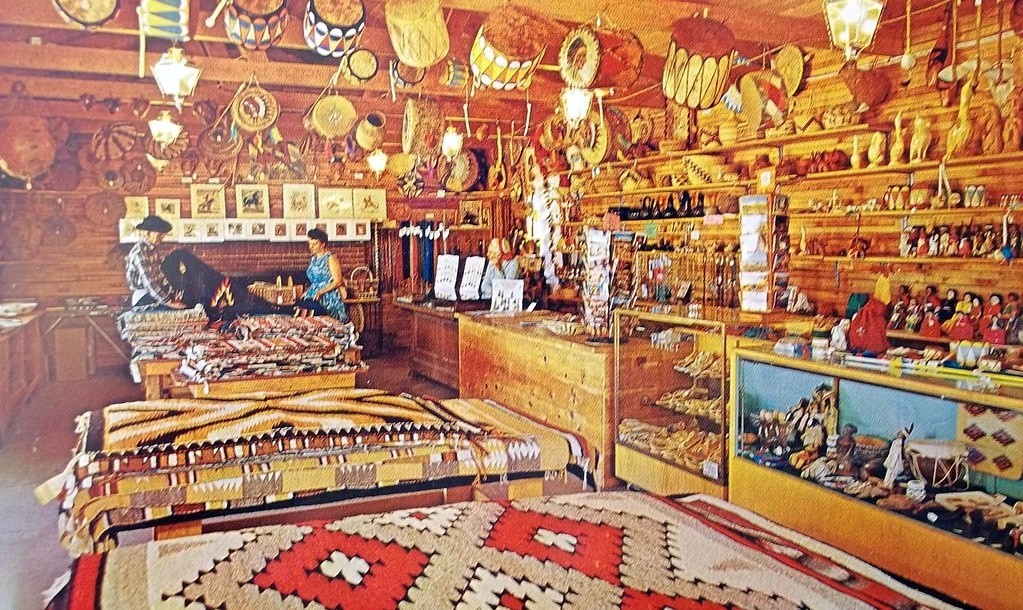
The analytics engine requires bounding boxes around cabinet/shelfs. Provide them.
[0,308,48,442]
[801,151,1022,347]
[614,307,817,503]
[403,303,460,393]
[584,122,883,221]
[456,312,692,491]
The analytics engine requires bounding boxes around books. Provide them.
[578,230,635,342]
[738,194,791,310]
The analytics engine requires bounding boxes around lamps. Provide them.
[823,0,886,69]
[148,46,204,114]
[368,148,387,184]
[127,96,152,120]
[148,113,185,154]
[440,120,464,163]
[191,96,216,127]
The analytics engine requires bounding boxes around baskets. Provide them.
[246,285,296,306]
[345,267,378,299]
[545,320,584,337]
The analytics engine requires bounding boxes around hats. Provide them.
[135,216,173,232]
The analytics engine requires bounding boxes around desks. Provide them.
[49,311,116,382]
[141,349,360,400]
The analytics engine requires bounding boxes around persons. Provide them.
[125,215,189,310]
[836,424,860,476]
[904,223,1023,265]
[294,229,349,324]
[886,285,1023,344]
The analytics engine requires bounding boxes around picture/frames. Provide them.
[124,196,149,218]
[353,188,387,222]
[318,187,353,219]
[154,197,182,219]
[282,181,316,219]
[235,182,270,219]
[189,183,226,219]
